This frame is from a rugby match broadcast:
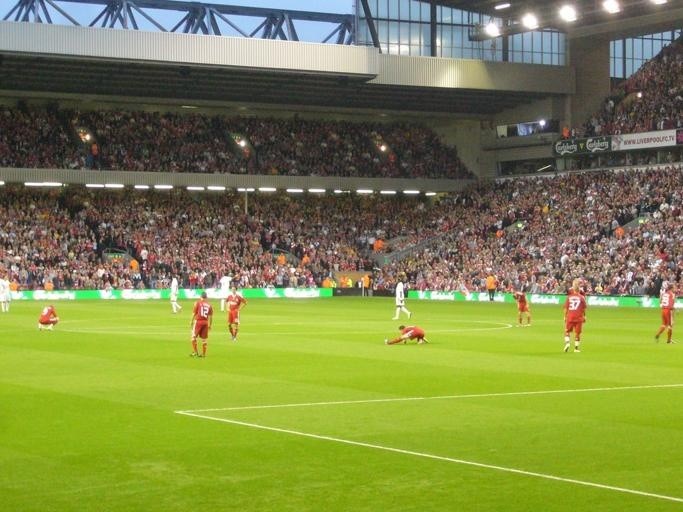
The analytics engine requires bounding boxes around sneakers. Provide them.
[563,343,581,353]
[392,311,412,321]
